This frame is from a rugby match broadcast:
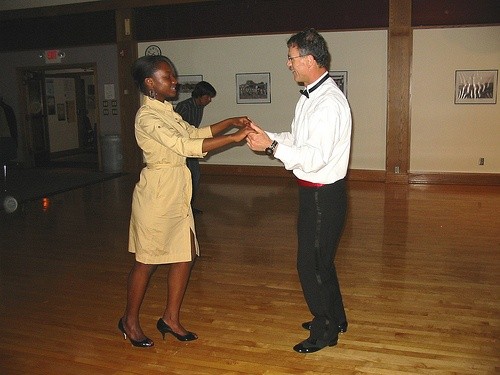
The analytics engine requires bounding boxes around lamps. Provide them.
[38,49,66,65]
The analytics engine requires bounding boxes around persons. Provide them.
[244,28,352,353]
[118,56,258,347]
[0,97,18,183]
[175,81,215,216]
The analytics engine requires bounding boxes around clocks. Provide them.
[144,44,162,57]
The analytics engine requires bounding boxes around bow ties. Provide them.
[299,73,329,98]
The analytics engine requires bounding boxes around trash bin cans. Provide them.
[100,132,122,174]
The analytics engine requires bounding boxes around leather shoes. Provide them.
[301,321,348,333]
[293,338,338,353]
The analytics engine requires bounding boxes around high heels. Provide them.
[156,318,197,342]
[118,318,154,347]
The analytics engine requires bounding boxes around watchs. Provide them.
[264,140,278,156]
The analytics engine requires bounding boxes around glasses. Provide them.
[288,53,311,61]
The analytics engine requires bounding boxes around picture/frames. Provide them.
[328,70,348,100]
[235,72,272,104]
[454,69,498,104]
[172,74,203,105]
